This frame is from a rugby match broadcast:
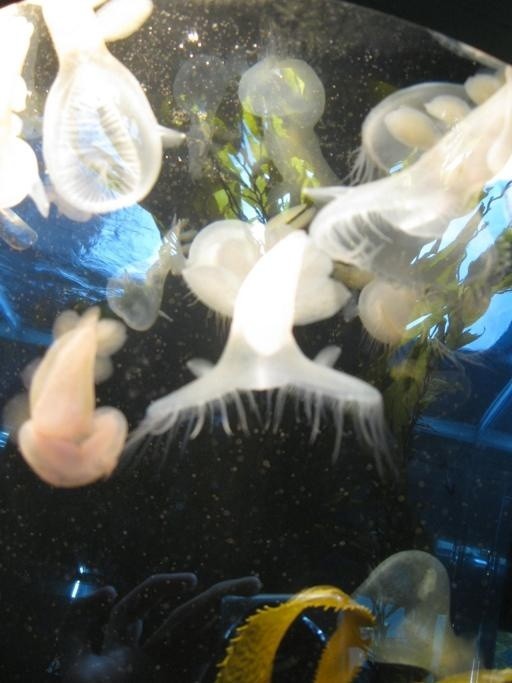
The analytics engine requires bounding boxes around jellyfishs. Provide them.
[330,542,484,683]
[0,0,512,489]
